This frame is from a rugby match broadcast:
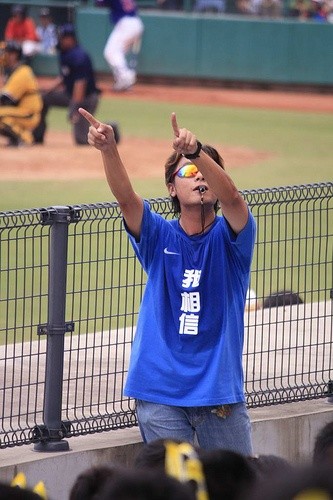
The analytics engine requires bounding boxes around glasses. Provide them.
[178,164,199,178]
